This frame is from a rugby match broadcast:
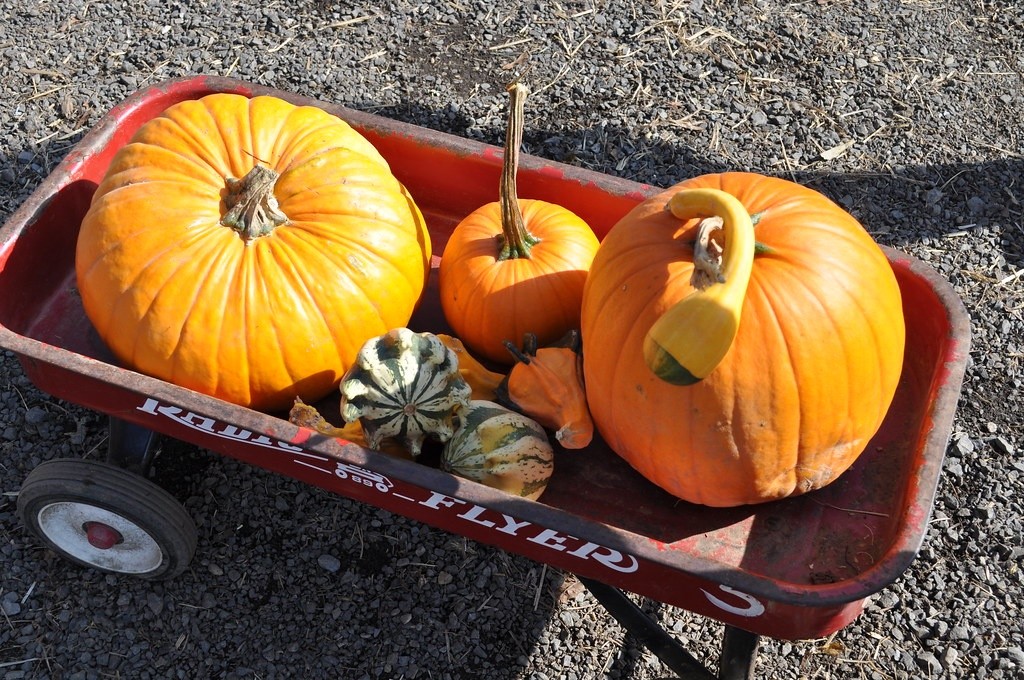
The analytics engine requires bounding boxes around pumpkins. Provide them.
[73,82,901,509]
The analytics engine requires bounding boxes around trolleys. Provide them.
[0,73,972,680]
